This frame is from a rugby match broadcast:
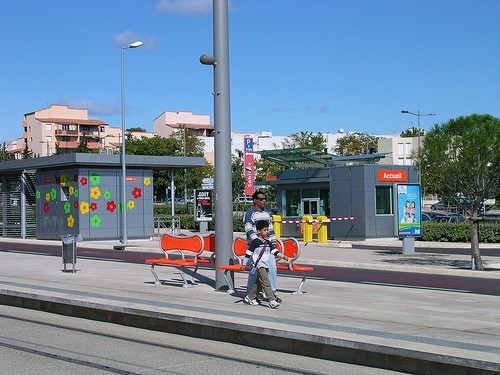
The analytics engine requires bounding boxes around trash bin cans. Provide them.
[61,234,77,264]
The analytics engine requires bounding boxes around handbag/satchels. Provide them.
[250,261,257,275]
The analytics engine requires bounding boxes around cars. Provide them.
[422,196,500,224]
[266,209,278,217]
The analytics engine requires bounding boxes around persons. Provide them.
[240,220,289,308]
[244,191,283,303]
[402,201,416,223]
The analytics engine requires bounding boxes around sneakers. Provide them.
[268,299,281,309]
[257,288,268,300]
[273,292,283,303]
[244,295,259,306]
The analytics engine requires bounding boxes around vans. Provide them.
[234,197,253,203]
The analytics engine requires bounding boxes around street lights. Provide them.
[120,40,144,251]
[401,109,437,190]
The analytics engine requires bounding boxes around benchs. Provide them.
[144,234,313,295]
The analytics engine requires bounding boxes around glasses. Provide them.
[257,197,268,202]
